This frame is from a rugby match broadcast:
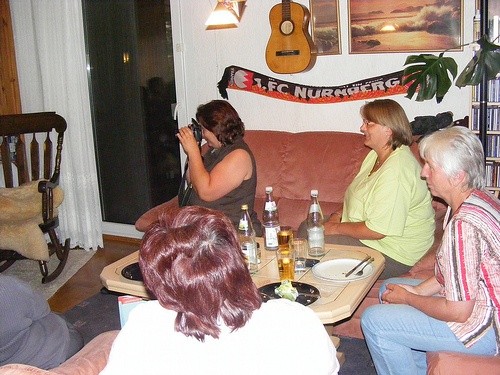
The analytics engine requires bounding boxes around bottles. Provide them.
[263,186,281,251]
[237,204,258,273]
[306,189,326,257]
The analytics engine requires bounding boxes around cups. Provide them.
[277,231,293,247]
[288,238,308,272]
[276,247,296,282]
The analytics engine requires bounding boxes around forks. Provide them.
[354,258,375,276]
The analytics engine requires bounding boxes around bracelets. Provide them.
[331,212,341,217]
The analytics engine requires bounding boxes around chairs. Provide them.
[0,112,71,284]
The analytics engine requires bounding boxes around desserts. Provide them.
[274,280,298,302]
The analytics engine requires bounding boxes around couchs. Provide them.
[425,351,500,375]
[0,330,121,375]
[132,130,453,338]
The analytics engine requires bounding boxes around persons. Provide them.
[323,98,436,280]
[0,273,84,370]
[360,126,500,375]
[106,205,341,375]
[175,99,257,226]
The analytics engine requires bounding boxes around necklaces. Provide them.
[376,151,390,167]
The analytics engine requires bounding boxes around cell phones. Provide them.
[294,257,320,267]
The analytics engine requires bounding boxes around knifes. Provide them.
[345,256,371,277]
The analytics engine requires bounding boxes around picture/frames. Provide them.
[347,0,464,55]
[308,0,342,55]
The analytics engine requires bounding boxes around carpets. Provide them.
[0,249,97,300]
[60,286,381,375]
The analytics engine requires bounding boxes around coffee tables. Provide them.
[102,232,385,364]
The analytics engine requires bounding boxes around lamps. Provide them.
[205,0,245,31]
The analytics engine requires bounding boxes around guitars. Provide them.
[265,0,317,74]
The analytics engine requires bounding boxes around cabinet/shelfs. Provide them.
[81,0,183,226]
[472,18,500,199]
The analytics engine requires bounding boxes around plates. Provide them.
[311,258,375,283]
[257,281,320,306]
